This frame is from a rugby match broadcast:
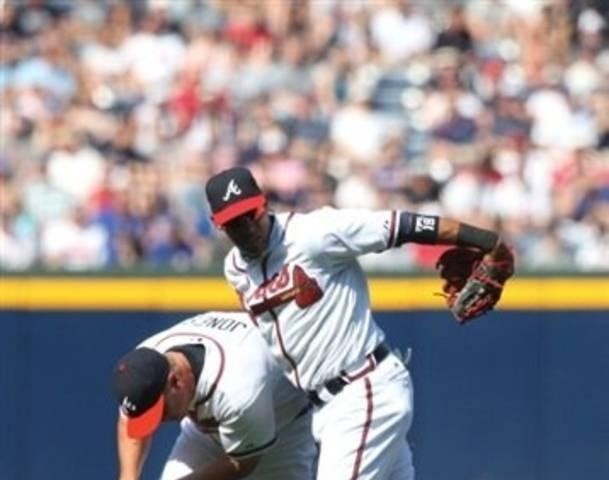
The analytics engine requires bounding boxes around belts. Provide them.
[307,342,391,408]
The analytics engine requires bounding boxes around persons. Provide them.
[0,0,609,278]
[206,165,515,479]
[112,307,319,480]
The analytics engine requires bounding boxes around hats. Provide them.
[206,166,266,227]
[113,347,171,438]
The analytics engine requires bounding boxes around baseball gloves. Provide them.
[436,239,514,326]
